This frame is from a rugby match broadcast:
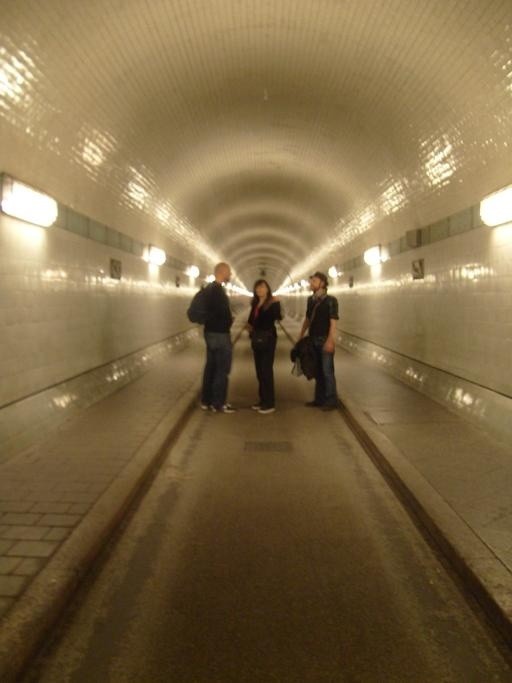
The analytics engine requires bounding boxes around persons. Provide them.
[187,262,237,413]
[299,272,339,411]
[248,279,283,413]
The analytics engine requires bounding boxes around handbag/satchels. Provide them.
[252,333,270,351]
[291,355,303,378]
[187,283,213,325]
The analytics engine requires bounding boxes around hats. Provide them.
[309,272,327,283]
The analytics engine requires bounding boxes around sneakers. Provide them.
[251,403,275,413]
[201,401,236,414]
[305,401,339,410]
